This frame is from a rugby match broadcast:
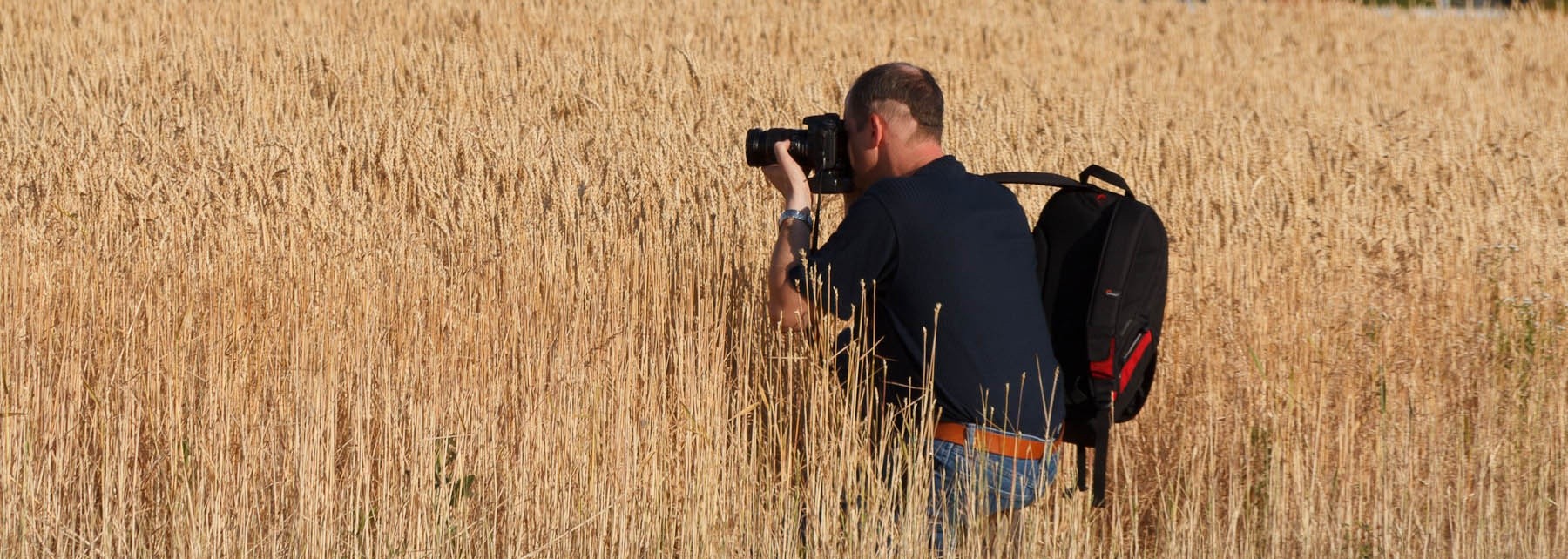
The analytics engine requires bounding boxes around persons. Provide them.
[761,62,1066,559]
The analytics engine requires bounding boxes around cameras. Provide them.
[745,112,854,195]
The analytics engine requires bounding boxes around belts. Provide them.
[930,422,1067,459]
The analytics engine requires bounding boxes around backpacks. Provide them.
[984,164,1170,447]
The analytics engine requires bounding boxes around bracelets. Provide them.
[778,210,813,233]
